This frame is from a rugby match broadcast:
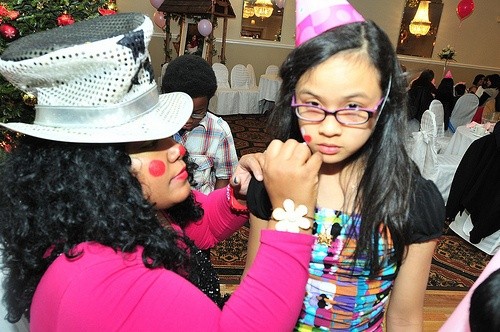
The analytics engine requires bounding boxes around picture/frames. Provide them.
[179,14,210,60]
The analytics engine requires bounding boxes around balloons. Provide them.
[198,19,212,36]
[150,0,165,9]
[153,11,166,29]
[455,0,474,21]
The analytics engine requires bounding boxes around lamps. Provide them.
[409,1,432,38]
[242,0,254,19]
[253,0,274,21]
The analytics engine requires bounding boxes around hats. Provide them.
[0,12,194,143]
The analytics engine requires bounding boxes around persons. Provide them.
[240,0,445,332]
[161,55,238,196]
[408,69,500,130]
[0,12,323,332]
[469,267,500,332]
[185,34,202,56]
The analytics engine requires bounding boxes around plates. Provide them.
[473,132,484,137]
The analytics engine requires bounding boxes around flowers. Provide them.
[0,0,118,160]
[438,45,458,68]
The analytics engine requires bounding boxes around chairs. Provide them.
[161,63,279,115]
[412,93,500,255]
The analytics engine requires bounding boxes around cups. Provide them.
[477,128,488,135]
[489,127,494,134]
[474,124,484,130]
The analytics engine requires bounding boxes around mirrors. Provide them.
[397,0,444,58]
[241,0,284,41]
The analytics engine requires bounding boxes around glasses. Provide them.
[290,95,386,126]
[191,105,209,119]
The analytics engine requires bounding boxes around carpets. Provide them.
[208,113,494,291]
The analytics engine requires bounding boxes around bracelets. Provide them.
[225,184,249,217]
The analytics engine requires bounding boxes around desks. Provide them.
[446,123,496,155]
[258,75,283,114]
